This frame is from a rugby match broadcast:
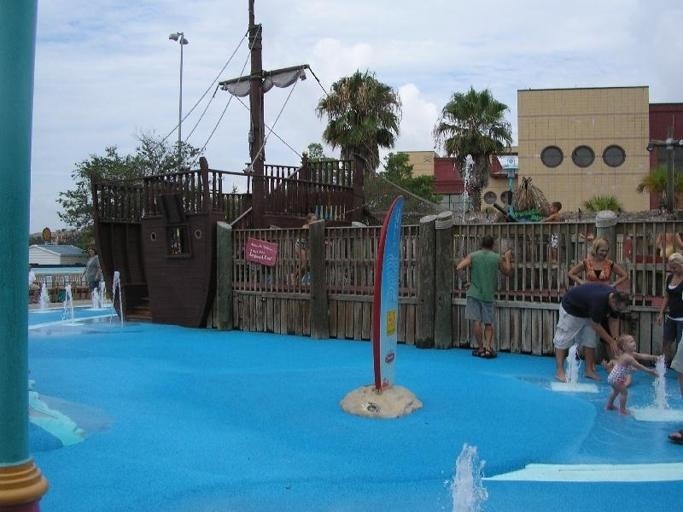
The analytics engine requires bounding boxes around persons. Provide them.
[454,234,512,359]
[28,263,36,305]
[654,252,682,363]
[293,213,317,276]
[653,231,682,259]
[604,332,665,415]
[665,328,682,443]
[550,280,633,384]
[622,234,633,264]
[539,200,562,265]
[566,237,629,373]
[79,246,104,309]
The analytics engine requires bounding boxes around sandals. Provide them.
[470,349,498,358]
[666,428,683,443]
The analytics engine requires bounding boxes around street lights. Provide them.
[168,30,189,172]
